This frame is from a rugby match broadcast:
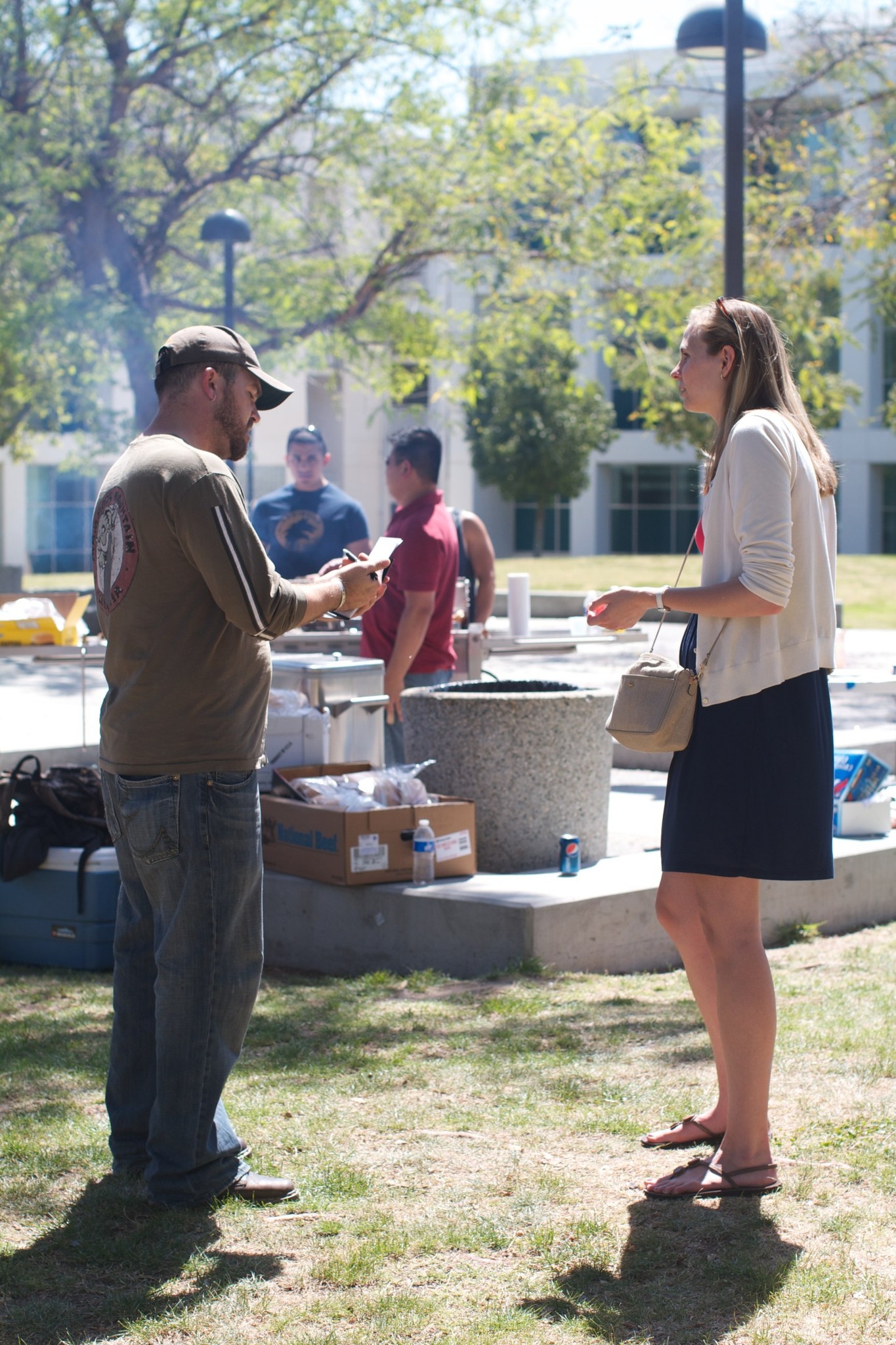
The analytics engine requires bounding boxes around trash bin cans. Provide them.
[400,673,615,869]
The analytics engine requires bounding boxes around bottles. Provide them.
[412,819,435,885]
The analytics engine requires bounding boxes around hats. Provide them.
[155,325,295,411]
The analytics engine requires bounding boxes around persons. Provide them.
[85,324,395,1206]
[583,295,845,1202]
[362,425,497,766]
[247,426,374,580]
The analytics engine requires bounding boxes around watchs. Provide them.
[655,585,672,614]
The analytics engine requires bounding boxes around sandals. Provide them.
[643,1154,783,1199]
[640,1114,725,1148]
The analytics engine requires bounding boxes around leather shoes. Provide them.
[236,1136,252,1160]
[213,1171,299,1204]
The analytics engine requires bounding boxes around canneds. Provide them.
[558,834,581,876]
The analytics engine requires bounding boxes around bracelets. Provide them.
[331,577,346,614]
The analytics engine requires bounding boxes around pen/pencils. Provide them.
[342,548,379,581]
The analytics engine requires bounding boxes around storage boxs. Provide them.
[834,747,893,838]
[1,846,121,970]
[0,591,91,645]
[243,655,475,884]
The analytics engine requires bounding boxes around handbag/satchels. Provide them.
[0,755,46,883]
[605,652,698,753]
[38,767,112,846]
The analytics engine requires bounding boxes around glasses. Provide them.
[287,427,321,444]
